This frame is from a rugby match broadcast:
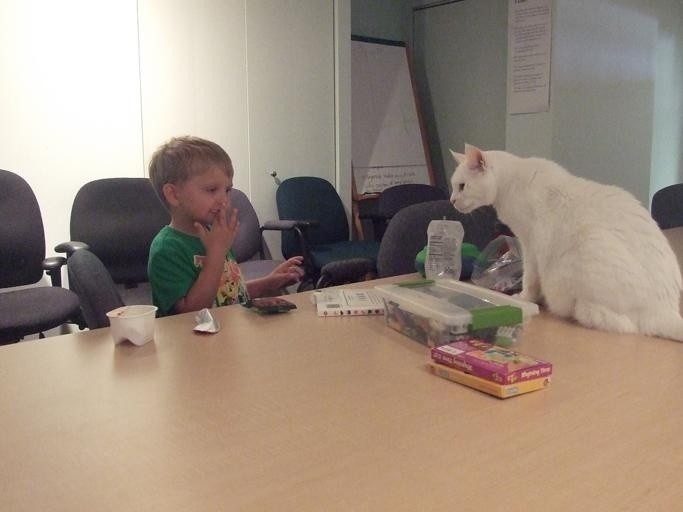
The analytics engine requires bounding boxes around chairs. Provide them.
[276,176,382,289]
[0,170,80,345]
[650,182,682,231]
[225,189,310,297]
[54,178,171,330]
[371,184,515,278]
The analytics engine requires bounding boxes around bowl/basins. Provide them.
[107,306,158,347]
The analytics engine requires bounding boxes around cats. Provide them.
[448,142,683,342]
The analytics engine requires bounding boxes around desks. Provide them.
[0,224,683,512]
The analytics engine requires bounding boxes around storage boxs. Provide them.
[374,274,541,353]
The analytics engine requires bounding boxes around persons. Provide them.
[146,134,304,318]
